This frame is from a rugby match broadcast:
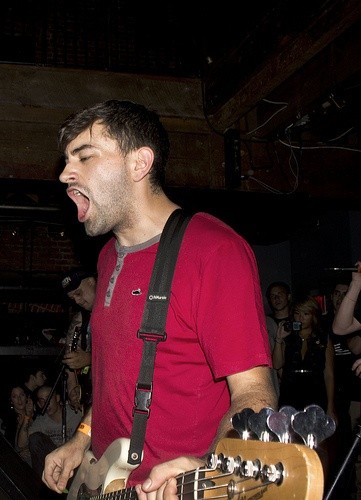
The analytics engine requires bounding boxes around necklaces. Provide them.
[299,331,311,343]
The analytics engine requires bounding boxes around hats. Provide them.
[61,265,96,294]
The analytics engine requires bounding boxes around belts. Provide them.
[292,369,311,373]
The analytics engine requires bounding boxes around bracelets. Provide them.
[77,423,91,438]
[275,340,283,344]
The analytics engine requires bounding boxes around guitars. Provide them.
[66,404,336,500]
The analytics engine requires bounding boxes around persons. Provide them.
[9,366,83,470]
[263,258,361,500]
[59,269,97,412]
[41,100,279,500]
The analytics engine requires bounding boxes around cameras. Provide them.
[283,320,302,332]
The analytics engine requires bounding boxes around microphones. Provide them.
[323,267,358,272]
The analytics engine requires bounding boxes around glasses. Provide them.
[293,310,314,320]
